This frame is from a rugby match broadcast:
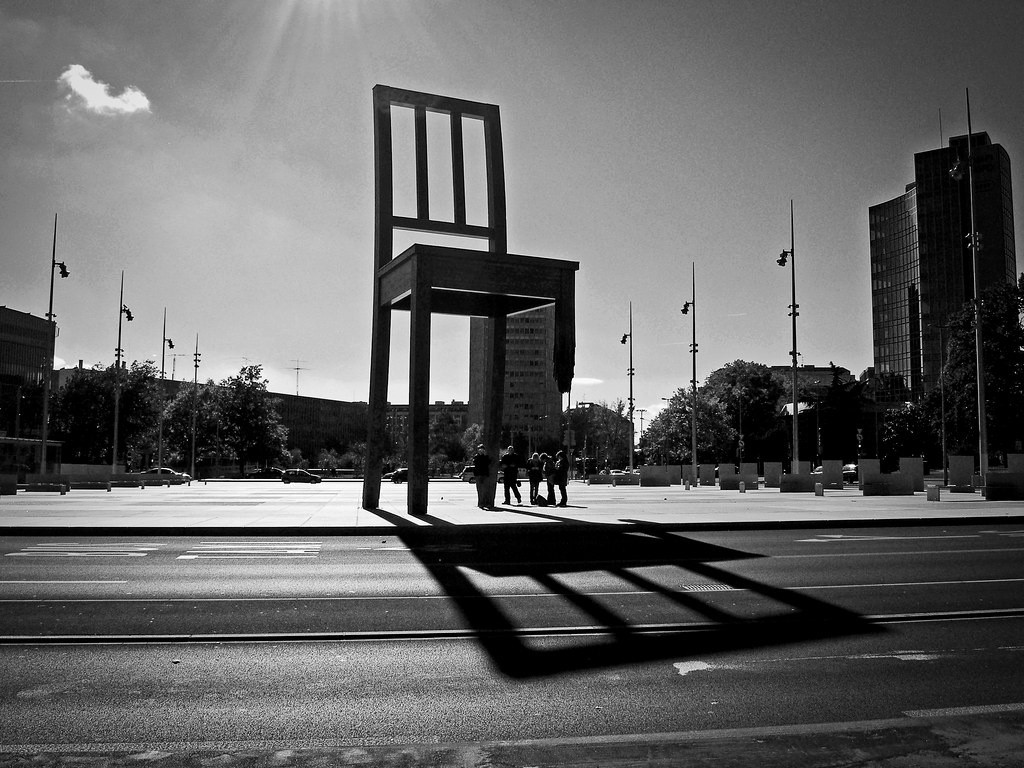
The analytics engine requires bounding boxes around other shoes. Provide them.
[518,496,521,503]
[502,501,510,505]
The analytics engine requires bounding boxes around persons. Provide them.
[606,465,610,475]
[393,469,403,484]
[498,445,521,505]
[525,449,569,507]
[472,443,496,510]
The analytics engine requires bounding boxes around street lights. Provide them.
[191,352,201,480]
[158,337,175,476]
[636,409,646,440]
[776,249,803,476]
[37,261,71,475]
[681,301,698,487]
[112,302,134,475]
[926,318,954,488]
[620,333,634,476]
[948,156,989,474]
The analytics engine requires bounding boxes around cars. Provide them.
[598,464,640,476]
[390,468,409,484]
[140,469,191,484]
[246,467,283,478]
[842,464,860,485]
[281,469,322,484]
[810,466,824,473]
[461,466,506,485]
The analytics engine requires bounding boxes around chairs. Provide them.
[362,84,580,516]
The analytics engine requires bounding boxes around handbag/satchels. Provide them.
[535,495,548,507]
[548,474,555,485]
[473,468,480,476]
[528,470,543,483]
